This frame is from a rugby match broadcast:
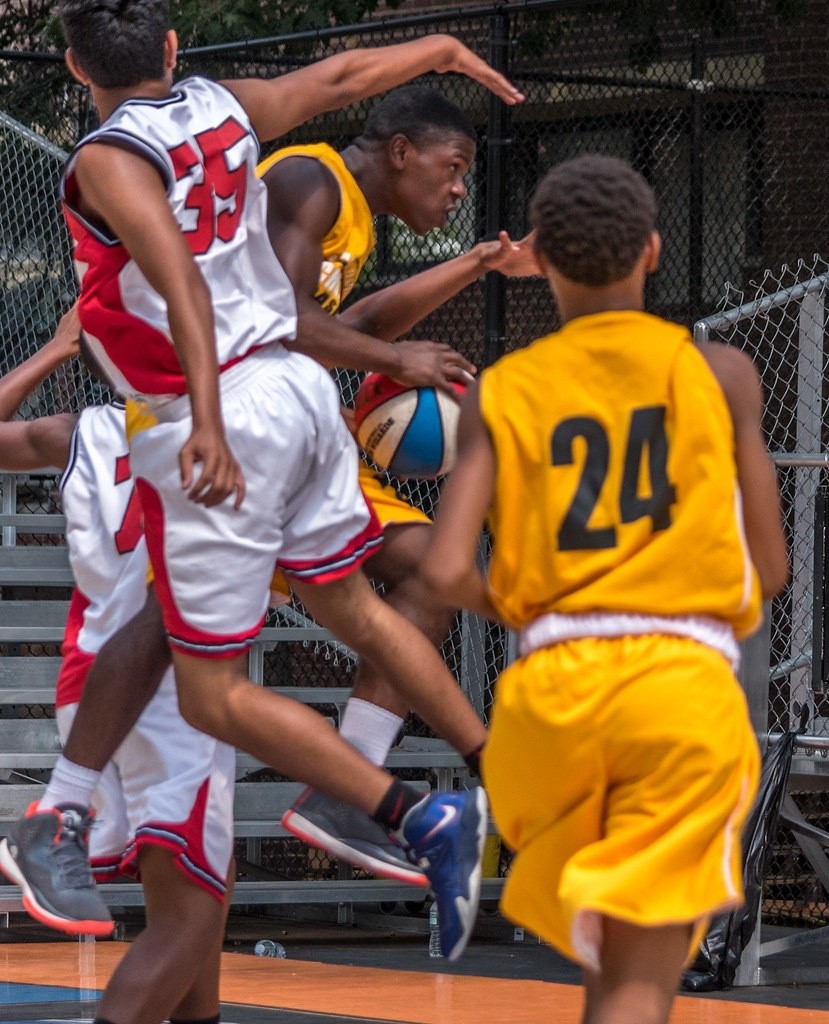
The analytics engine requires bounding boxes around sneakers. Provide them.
[0,800,115,937]
[392,785,489,961]
[281,783,430,888]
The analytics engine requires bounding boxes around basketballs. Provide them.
[352,362,477,480]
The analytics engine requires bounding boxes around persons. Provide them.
[0,83,549,939]
[408,153,792,1024]
[0,291,246,1024]
[55,1,526,964]
